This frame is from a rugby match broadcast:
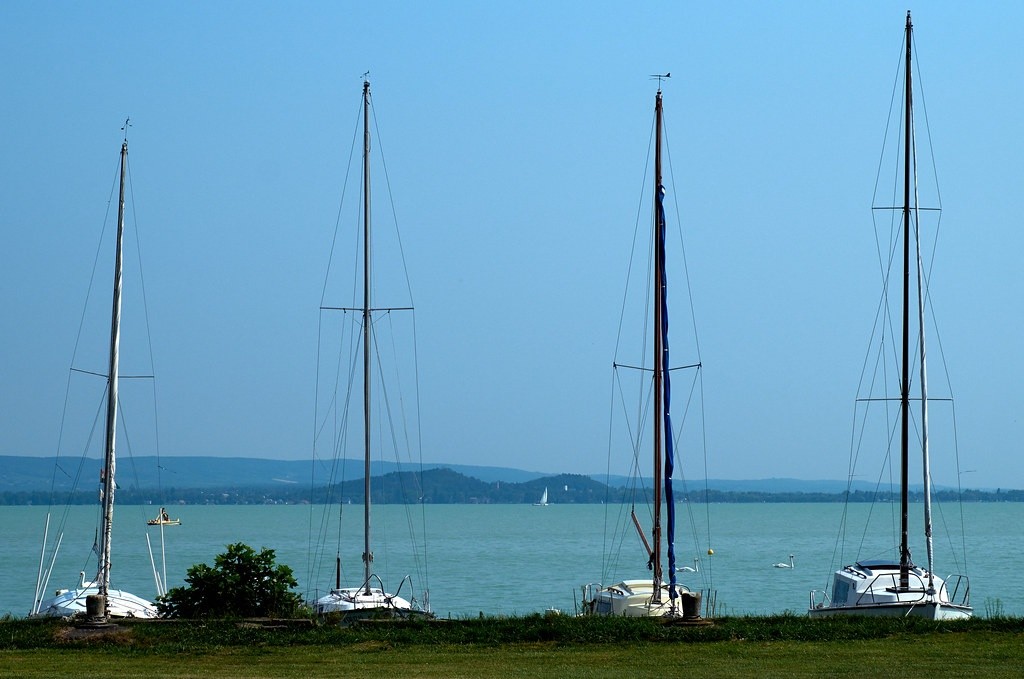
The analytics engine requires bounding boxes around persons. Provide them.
[163,512,169,521]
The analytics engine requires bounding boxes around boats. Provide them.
[146,518,180,526]
[18,118,175,622]
[299,71,432,621]
[807,8,979,626]
[578,69,710,622]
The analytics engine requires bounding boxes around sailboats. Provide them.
[532,486,549,506]
